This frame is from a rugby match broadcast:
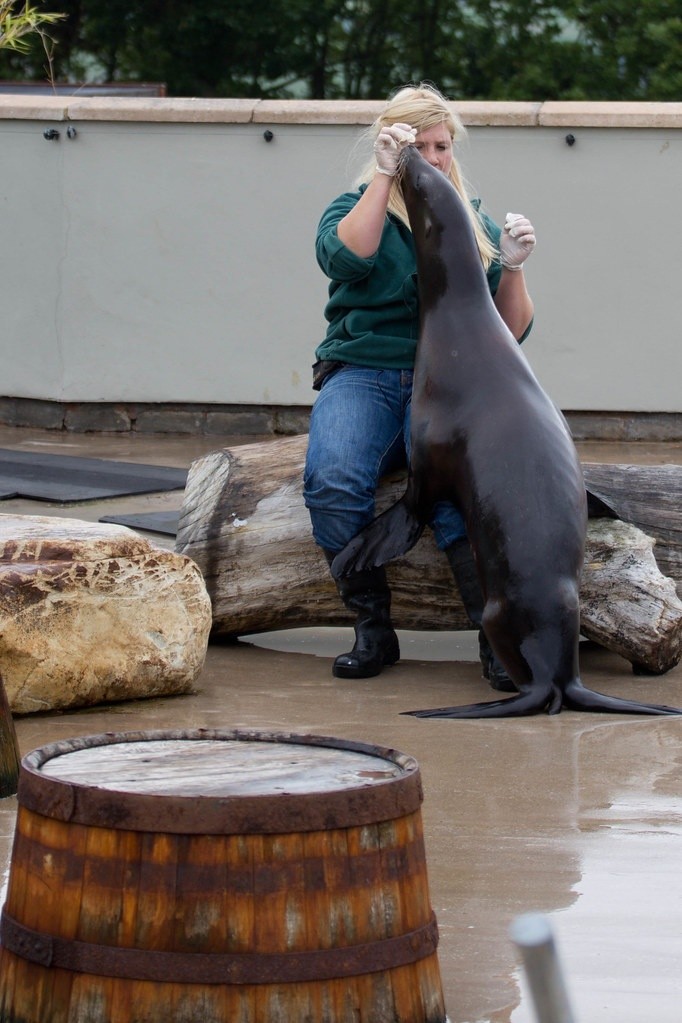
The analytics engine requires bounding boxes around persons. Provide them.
[299,81,537,693]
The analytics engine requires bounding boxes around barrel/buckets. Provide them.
[1,726,446,1023]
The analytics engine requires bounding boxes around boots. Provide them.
[444,535,522,693]
[318,541,400,680]
